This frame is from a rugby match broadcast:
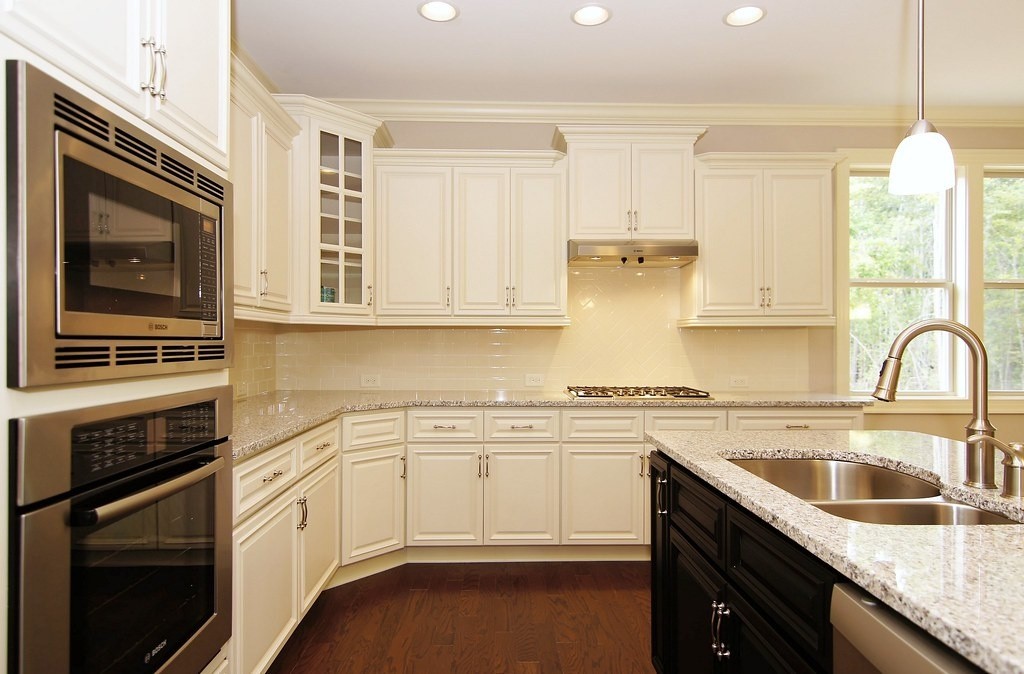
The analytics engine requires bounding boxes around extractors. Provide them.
[568,239,700,267]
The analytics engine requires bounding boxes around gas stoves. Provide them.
[563,384,714,403]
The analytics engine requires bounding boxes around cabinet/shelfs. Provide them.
[408,408,560,546]
[0,0,232,173]
[224,73,294,318]
[287,103,378,326]
[233,416,345,674]
[724,403,868,432]
[566,137,695,237]
[341,410,406,566]
[678,160,832,318]
[379,156,451,314]
[561,409,725,547]
[63,159,174,244]
[451,155,567,314]
[648,447,836,674]
[72,473,217,550]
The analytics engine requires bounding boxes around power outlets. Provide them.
[525,373,544,387]
[360,372,380,387]
[729,375,751,389]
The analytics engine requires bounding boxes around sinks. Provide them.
[811,503,1024,531]
[717,451,943,500]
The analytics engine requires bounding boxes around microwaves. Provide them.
[5,54,235,388]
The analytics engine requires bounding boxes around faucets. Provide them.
[869,319,1024,499]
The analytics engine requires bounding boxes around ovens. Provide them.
[10,387,233,674]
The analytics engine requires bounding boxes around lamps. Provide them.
[888,0,955,195]
